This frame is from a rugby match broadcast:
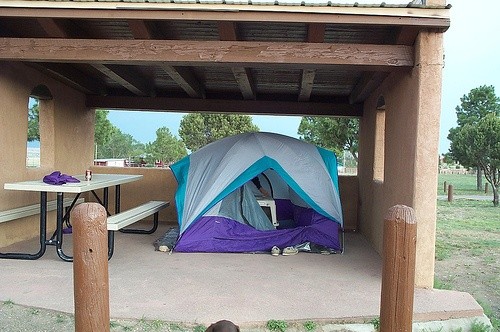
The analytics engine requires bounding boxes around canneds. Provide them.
[86,170,92,180]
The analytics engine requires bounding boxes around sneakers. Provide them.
[282,246,298,255]
[271,245,281,255]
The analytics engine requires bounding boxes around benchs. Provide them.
[0,198,85,260]
[57,201,170,262]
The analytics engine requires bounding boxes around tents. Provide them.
[169,132,344,255]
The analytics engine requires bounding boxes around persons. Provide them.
[251,177,270,197]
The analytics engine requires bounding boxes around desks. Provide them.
[0,175,159,262]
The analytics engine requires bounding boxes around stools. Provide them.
[255,196,279,230]
